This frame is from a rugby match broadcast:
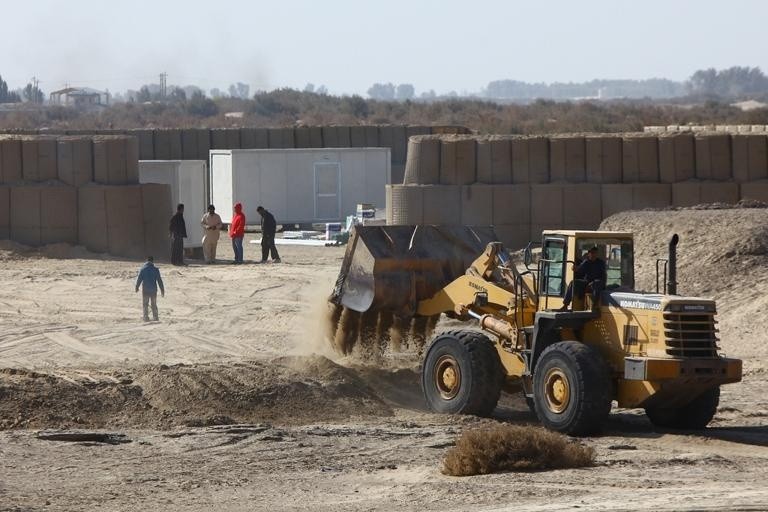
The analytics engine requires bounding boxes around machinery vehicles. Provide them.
[327,223,742,437]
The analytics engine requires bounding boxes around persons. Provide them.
[229,202,246,265]
[256,206,281,264]
[200,204,223,264]
[559,246,606,312]
[169,204,188,267]
[134,256,165,323]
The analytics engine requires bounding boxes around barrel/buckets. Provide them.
[326,223,341,240]
